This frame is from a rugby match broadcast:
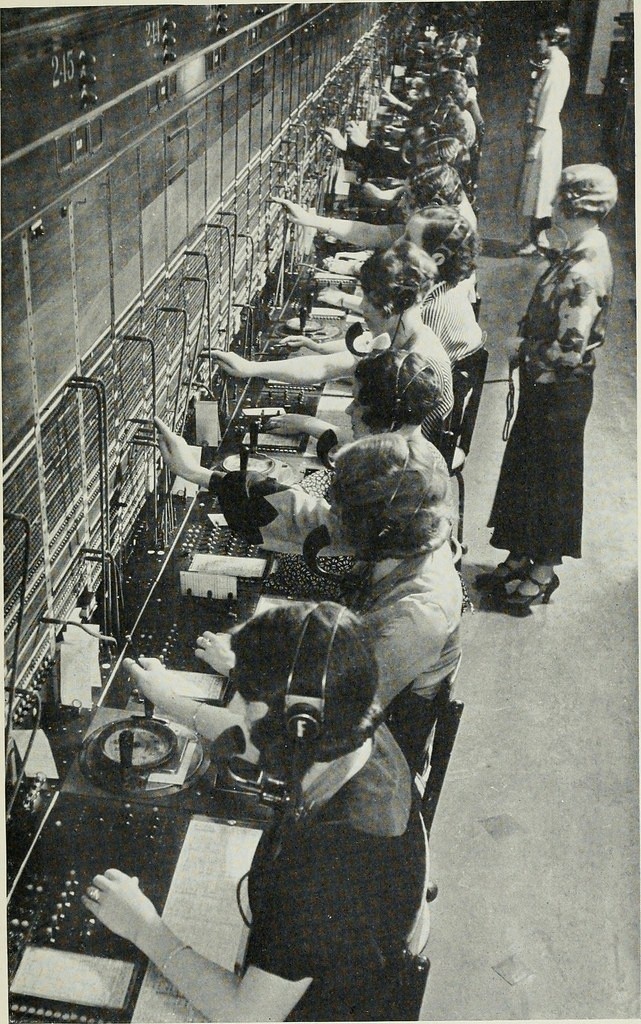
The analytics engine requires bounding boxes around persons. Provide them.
[272,12,484,315]
[81,601,426,1022]
[280,204,482,369]
[513,19,571,258]
[154,415,463,711]
[201,240,454,445]
[265,348,475,616]
[475,163,618,612]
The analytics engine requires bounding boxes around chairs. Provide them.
[401,883,438,1021]
[413,688,464,845]
[450,347,489,554]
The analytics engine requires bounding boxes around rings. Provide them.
[89,888,100,900]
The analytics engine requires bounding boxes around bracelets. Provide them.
[192,703,206,738]
[160,945,193,974]
[323,217,336,234]
[340,294,350,306]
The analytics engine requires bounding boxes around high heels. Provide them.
[473,555,559,616]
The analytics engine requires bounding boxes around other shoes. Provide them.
[513,239,549,255]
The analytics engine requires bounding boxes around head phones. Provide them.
[382,280,418,316]
[429,100,456,134]
[283,600,346,746]
[558,190,580,219]
[550,20,565,45]
[430,217,473,266]
[370,439,436,545]
[432,172,461,206]
[387,353,431,425]
